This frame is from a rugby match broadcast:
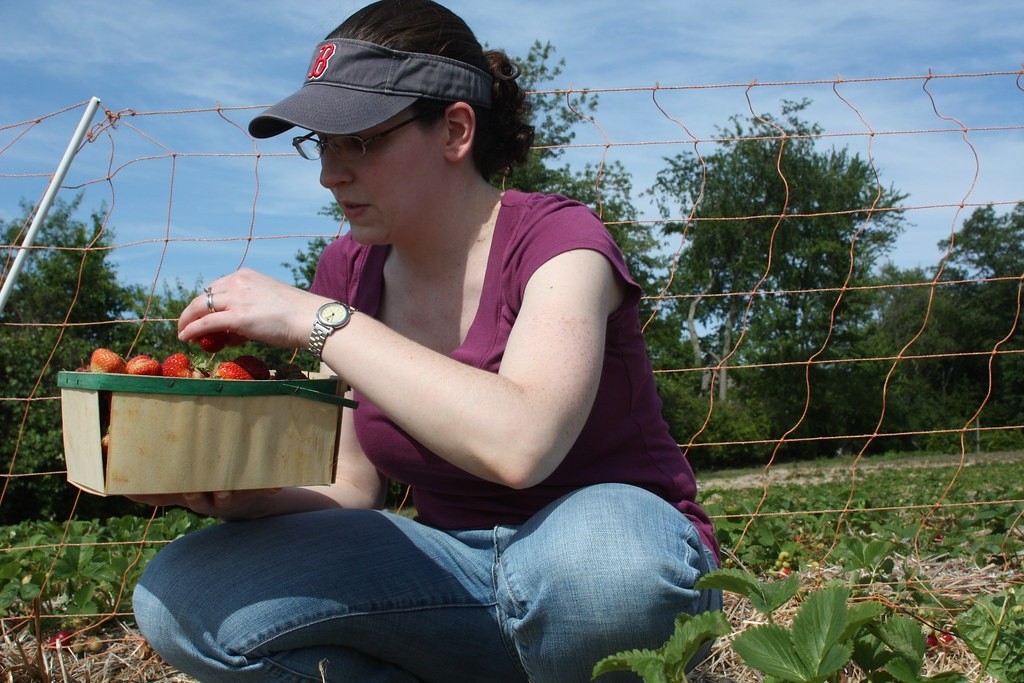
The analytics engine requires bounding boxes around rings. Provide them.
[204,286,216,313]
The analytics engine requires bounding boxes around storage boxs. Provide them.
[55,370,360,498]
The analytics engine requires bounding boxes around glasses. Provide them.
[292,113,424,160]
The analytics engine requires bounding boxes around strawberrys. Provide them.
[71,327,311,462]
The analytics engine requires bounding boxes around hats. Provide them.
[248,38,494,139]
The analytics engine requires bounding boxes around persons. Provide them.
[132,0,726,683]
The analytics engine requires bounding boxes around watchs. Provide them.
[308,300,357,361]
